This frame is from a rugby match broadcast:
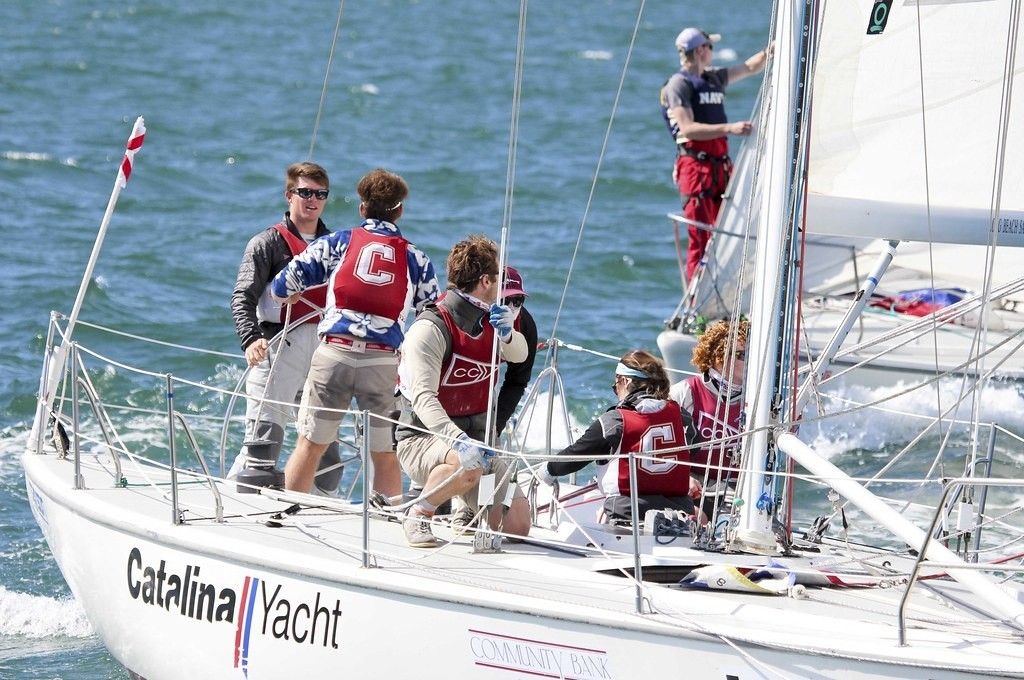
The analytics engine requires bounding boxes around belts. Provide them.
[327,336,395,351]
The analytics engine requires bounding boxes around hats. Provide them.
[675,27,721,52]
[501,266,529,299]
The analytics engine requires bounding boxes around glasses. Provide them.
[500,296,525,308]
[478,269,505,283]
[611,379,628,396]
[290,187,329,200]
[729,350,745,361]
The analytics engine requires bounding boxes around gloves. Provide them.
[488,303,514,339]
[451,433,495,471]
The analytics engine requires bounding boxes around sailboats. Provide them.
[20,1,1024,680]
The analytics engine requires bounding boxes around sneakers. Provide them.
[451,499,479,535]
[402,506,437,547]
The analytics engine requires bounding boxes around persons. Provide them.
[230,162,343,500]
[394,234,532,547]
[496,266,538,439]
[668,319,748,523]
[270,170,441,506]
[659,27,775,287]
[548,350,696,524]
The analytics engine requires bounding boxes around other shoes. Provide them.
[370,491,394,517]
[723,313,747,322]
[689,315,707,335]
